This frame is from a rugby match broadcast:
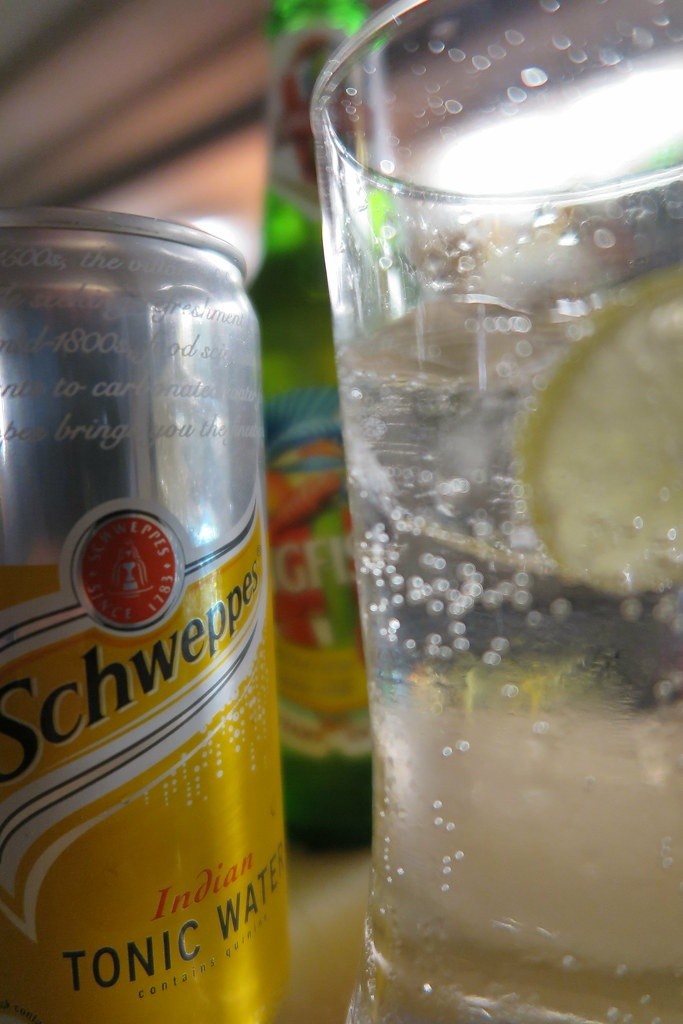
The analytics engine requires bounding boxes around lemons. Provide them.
[525,269,682,594]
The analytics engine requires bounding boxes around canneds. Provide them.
[0,208,292,1024]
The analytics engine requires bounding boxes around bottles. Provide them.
[250,0,478,849]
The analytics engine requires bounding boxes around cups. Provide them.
[308,0,683,1024]
